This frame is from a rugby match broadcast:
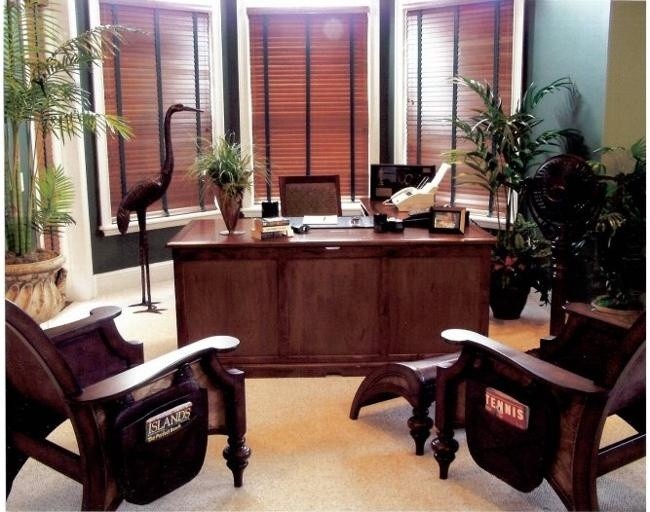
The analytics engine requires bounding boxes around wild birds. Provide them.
[117,104,204,315]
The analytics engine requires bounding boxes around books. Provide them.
[254,217,290,227]
[251,228,288,240]
[254,223,288,233]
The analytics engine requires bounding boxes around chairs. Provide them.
[164,195,496,377]
[6,299,251,510]
[276,173,343,218]
[434,300,645,512]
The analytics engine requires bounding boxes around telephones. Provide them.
[391,183,439,212]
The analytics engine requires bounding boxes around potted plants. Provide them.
[5,1,155,325]
[187,134,277,236]
[438,71,584,320]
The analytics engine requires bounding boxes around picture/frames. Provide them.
[429,207,466,235]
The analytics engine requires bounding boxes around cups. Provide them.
[262,201,278,219]
[389,222,404,232]
[373,222,387,232]
